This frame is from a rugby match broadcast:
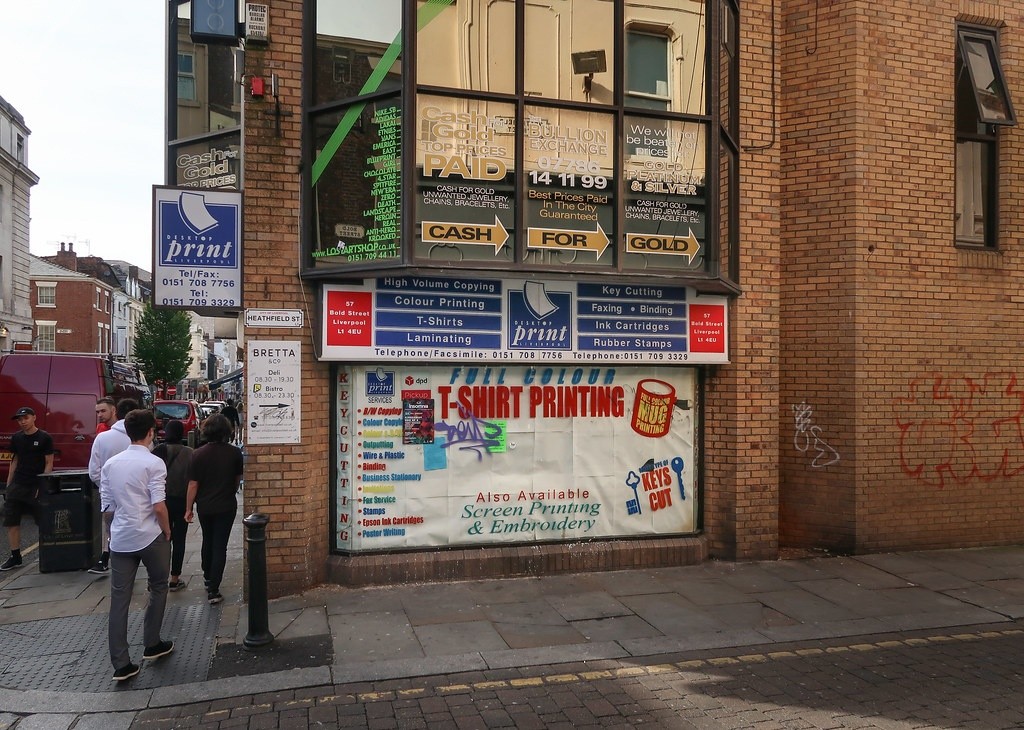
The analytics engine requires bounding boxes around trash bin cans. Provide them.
[36,469,103,573]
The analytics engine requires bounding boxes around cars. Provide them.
[201,401,229,417]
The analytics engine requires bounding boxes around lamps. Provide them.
[571,49,607,94]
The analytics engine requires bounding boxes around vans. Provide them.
[153,397,205,447]
[0,348,152,493]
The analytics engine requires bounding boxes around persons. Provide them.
[100,409,174,680]
[183,413,244,603]
[0,406,55,571]
[220,398,243,445]
[88,398,194,593]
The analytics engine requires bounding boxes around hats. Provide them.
[166,420,183,436]
[11,407,34,420]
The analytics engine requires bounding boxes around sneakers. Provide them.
[112,657,140,679]
[0,556,23,570]
[169,579,185,591]
[204,580,210,589]
[88,561,109,574]
[143,640,174,659]
[207,592,224,604]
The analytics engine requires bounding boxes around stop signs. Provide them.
[167,386,176,395]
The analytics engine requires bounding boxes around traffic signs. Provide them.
[525,221,610,262]
[623,226,702,266]
[420,212,510,258]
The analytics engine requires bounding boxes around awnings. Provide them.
[209,367,244,390]
